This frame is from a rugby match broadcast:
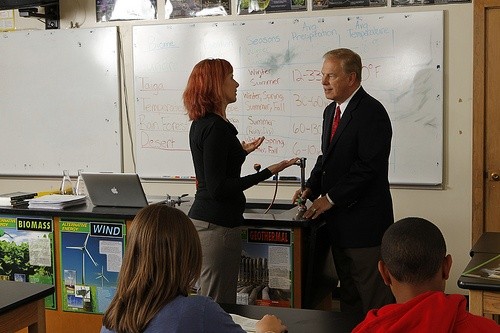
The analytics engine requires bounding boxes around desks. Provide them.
[457,232,500,325]
[0,199,171,316]
[0,280,55,333]
[216,302,367,333]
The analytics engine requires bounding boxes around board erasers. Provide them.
[279,176,297,181]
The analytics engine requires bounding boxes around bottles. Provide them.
[76,168,89,198]
[60,168,75,195]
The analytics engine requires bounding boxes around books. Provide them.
[0,191,86,210]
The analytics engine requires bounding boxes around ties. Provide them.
[330,106,341,142]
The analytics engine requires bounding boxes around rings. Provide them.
[312,208,316,212]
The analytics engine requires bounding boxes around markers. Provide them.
[175,175,195,178]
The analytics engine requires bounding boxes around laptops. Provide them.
[79,171,166,206]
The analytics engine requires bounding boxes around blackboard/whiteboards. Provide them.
[0,24,123,179]
[129,8,448,193]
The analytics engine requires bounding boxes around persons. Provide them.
[100,204,287,333]
[352,217,500,333]
[180,58,300,308]
[290,48,395,313]
